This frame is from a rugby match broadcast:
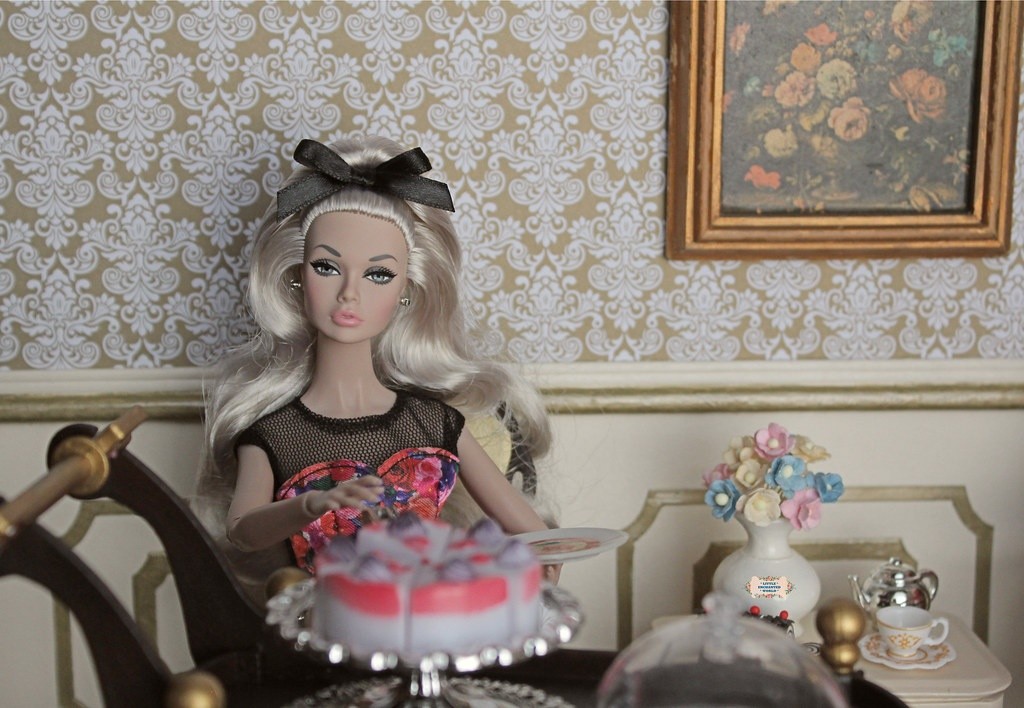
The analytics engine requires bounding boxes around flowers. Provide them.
[704,422,844,532]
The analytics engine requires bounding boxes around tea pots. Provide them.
[846,556,939,632]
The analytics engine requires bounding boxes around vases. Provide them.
[710,512,820,639]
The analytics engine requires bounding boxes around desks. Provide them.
[653,613,1012,708]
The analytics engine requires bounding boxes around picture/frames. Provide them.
[662,0,1024,260]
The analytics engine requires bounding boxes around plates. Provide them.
[509,528,629,564]
[858,631,957,670]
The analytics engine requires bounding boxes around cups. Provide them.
[875,606,949,658]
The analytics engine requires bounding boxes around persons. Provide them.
[192,132,561,622]
